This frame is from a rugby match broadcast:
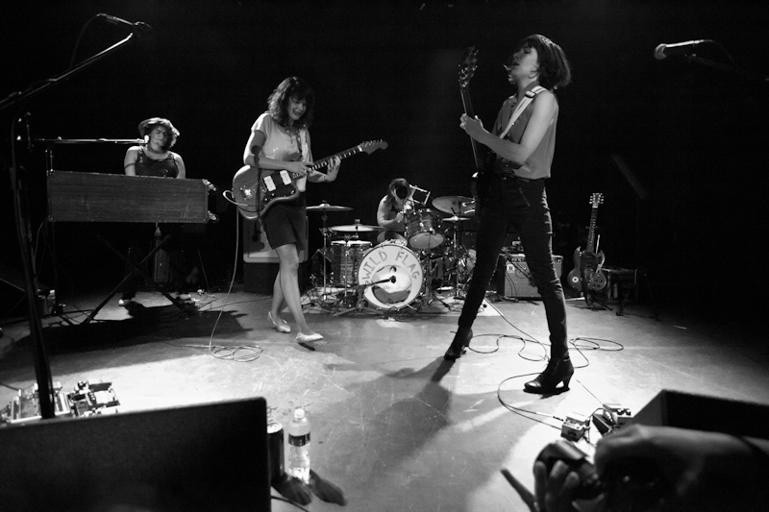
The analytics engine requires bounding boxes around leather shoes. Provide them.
[118,298,132,307]
[295,327,324,343]
[264,309,290,334]
[175,290,191,302]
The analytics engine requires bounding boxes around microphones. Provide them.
[144,135,151,145]
[654,39,713,60]
[96,11,152,40]
[390,265,396,284]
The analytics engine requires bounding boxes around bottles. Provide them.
[260,406,284,484]
[35,288,47,316]
[47,288,57,313]
[287,409,313,482]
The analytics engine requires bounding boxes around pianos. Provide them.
[47,170,212,223]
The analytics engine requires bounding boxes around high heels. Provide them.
[524,356,574,393]
[445,327,474,359]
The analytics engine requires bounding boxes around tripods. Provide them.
[301,217,345,310]
[415,235,451,314]
[333,277,388,319]
[428,222,487,308]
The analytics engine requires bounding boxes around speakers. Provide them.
[243,210,276,295]
[0,397,272,512]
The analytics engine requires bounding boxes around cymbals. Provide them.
[433,197,476,216]
[307,206,351,212]
[330,225,379,233]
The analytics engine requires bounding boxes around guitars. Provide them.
[461,48,495,225]
[231,140,388,220]
[568,192,607,291]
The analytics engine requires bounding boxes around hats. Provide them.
[138,117,181,148]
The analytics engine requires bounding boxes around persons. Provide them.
[377,178,415,247]
[443,33,574,395]
[242,75,341,343]
[118,117,197,309]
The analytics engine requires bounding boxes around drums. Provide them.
[356,239,426,309]
[404,209,443,249]
[332,240,371,286]
